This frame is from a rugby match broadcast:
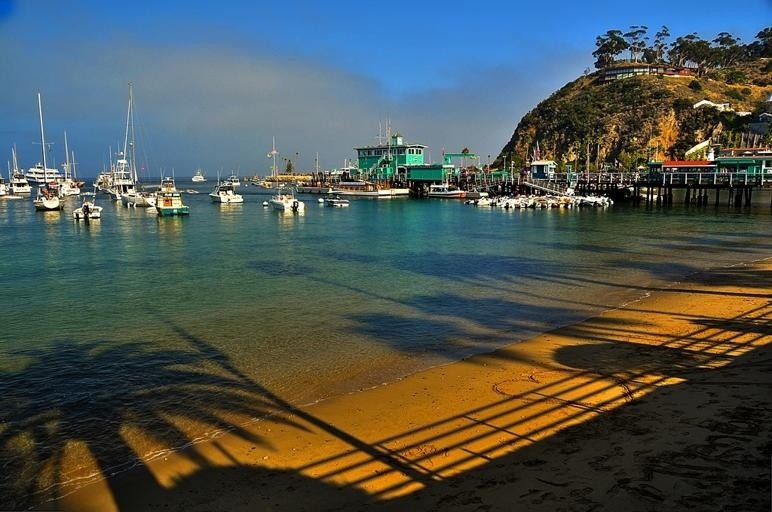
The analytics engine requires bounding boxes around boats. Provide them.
[427,185,466,198]
